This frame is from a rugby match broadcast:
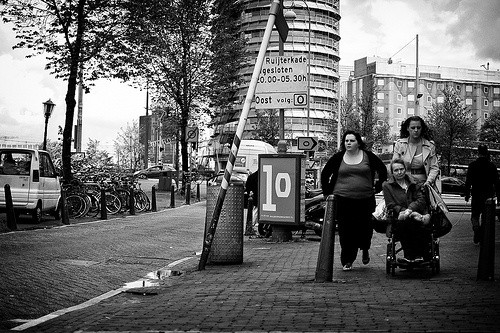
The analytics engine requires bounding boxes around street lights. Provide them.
[282,0,312,161]
[145,91,157,168]
[387,33,424,116]
[42,98,56,151]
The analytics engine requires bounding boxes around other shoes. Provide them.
[362,250,370,265]
[397,257,423,264]
[472,226,480,244]
[342,263,352,271]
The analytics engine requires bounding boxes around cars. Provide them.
[441,177,466,194]
[120,165,177,179]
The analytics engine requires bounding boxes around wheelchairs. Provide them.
[385,184,442,277]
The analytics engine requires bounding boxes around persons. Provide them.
[391,115,439,187]
[464,144,500,244]
[321,130,387,271]
[383,158,431,267]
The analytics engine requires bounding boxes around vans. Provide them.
[232,139,276,173]
[0,147,63,223]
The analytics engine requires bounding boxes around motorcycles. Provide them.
[257,188,327,237]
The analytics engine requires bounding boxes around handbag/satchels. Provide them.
[425,180,448,214]
[431,206,453,238]
[372,199,389,221]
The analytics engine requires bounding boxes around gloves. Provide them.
[398,209,412,221]
[409,212,422,221]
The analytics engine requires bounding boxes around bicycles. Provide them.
[64,174,150,218]
